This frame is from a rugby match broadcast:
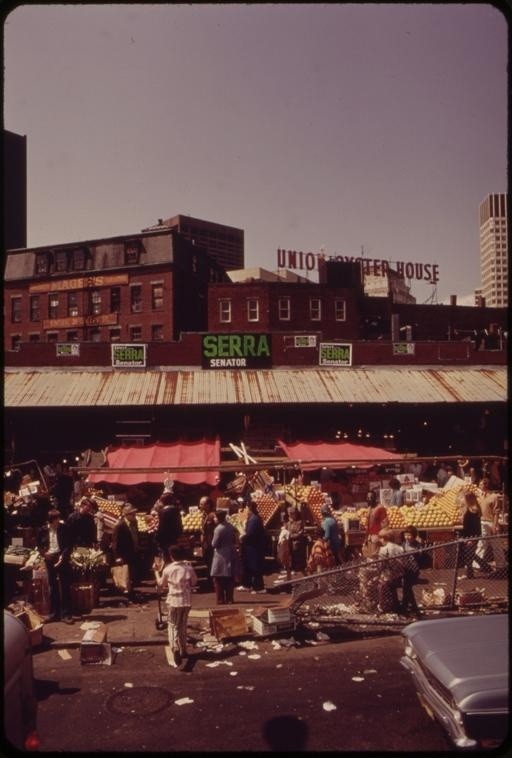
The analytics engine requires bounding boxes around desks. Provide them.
[345,525,464,570]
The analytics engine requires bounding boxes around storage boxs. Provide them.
[14,610,44,646]
[80,622,111,666]
[209,605,296,642]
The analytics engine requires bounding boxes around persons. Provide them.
[1,461,509,665]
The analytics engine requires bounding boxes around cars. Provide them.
[399,613,510,754]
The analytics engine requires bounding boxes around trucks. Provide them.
[2,610,39,755]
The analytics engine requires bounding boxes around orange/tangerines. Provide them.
[180,510,204,533]
[386,484,460,528]
[135,512,149,533]
[93,497,121,518]
